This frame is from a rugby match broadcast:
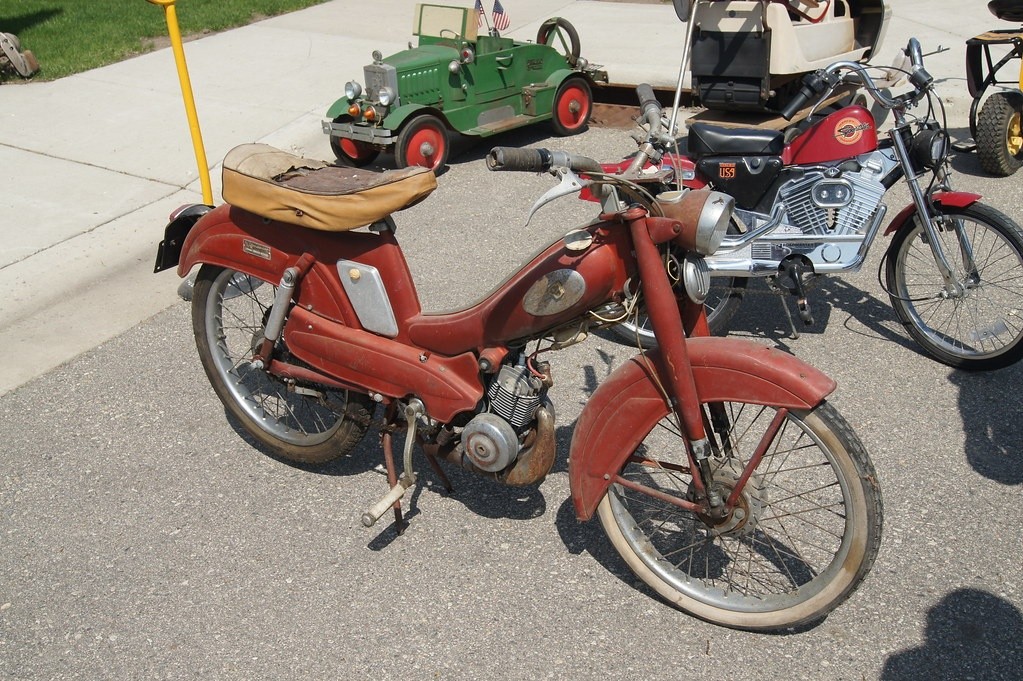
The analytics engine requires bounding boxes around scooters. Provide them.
[952,0,1023,175]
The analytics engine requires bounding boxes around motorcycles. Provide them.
[576,37,1023,373]
[150,84,883,637]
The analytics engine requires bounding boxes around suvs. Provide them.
[319,1,595,177]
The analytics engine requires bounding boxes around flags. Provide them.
[492,0,510,31]
[474,0,484,29]
